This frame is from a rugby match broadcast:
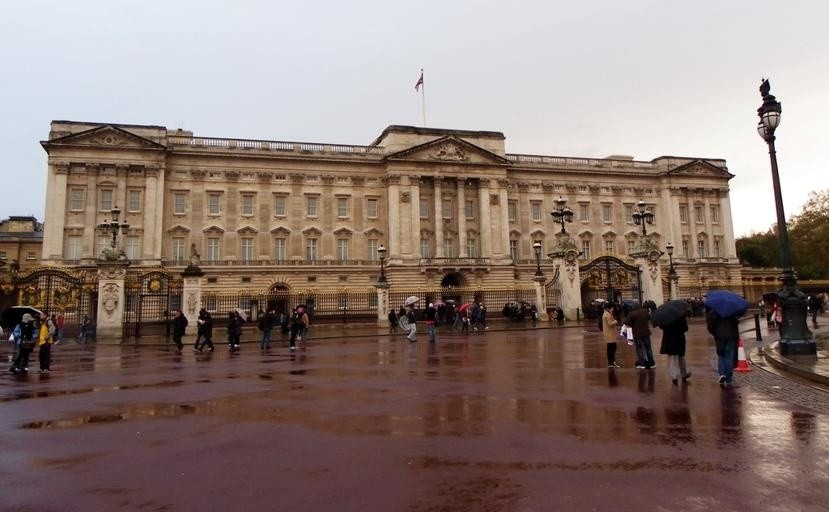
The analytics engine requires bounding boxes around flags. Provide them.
[415,73,423,92]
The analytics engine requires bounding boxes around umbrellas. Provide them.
[703,291,749,318]
[405,296,419,307]
[0,306,43,328]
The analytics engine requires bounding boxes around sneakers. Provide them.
[607,362,734,385]
[175,345,300,352]
[8,368,52,376]
[388,326,490,344]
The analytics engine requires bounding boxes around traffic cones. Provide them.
[731,337,753,372]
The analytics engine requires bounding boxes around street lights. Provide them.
[531,242,543,276]
[632,198,663,255]
[94,205,131,262]
[549,192,578,253]
[375,243,388,282]
[754,74,820,356]
[665,241,681,278]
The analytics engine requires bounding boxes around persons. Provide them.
[192,305,310,353]
[502,302,537,322]
[589,297,739,385]
[7,311,97,373]
[551,308,564,321]
[173,310,188,352]
[389,299,489,343]
[757,292,826,328]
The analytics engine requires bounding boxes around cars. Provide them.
[7,304,44,330]
[501,296,643,318]
